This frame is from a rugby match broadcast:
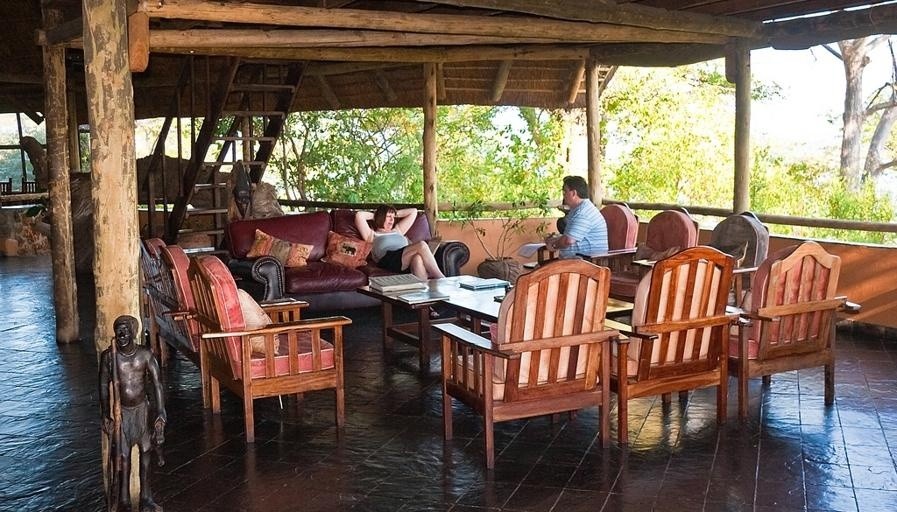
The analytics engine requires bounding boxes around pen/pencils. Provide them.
[549,232,555,239]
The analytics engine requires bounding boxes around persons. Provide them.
[98,314,169,512]
[355,202,445,279]
[530,175,611,272]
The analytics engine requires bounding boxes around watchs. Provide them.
[552,240,558,249]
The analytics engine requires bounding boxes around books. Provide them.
[514,243,544,256]
[367,273,427,293]
[396,290,450,304]
[458,276,510,290]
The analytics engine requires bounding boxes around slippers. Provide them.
[429,307,440,319]
